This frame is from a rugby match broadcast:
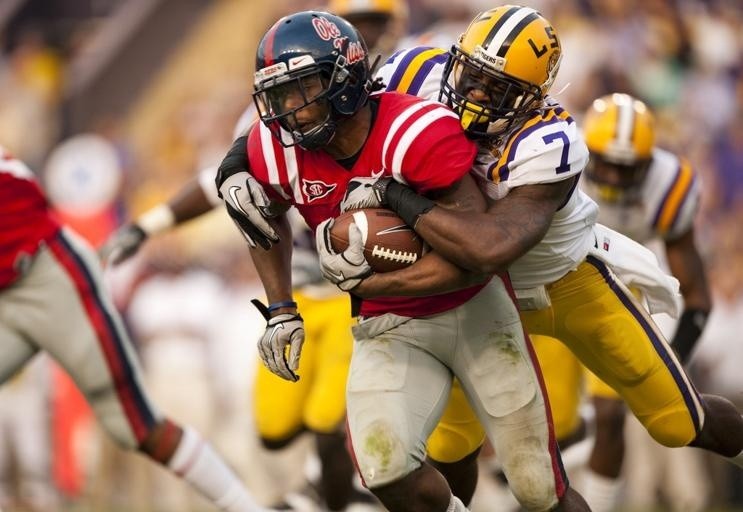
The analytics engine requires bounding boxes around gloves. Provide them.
[250,298,305,382]
[315,217,375,293]
[340,171,437,231]
[215,135,282,252]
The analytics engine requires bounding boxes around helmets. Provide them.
[250,10,386,154]
[437,4,563,141]
[578,93,655,202]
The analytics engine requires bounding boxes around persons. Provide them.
[2,0,743,512]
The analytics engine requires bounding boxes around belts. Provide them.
[351,312,412,342]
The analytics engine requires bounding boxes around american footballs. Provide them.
[330,207,432,273]
